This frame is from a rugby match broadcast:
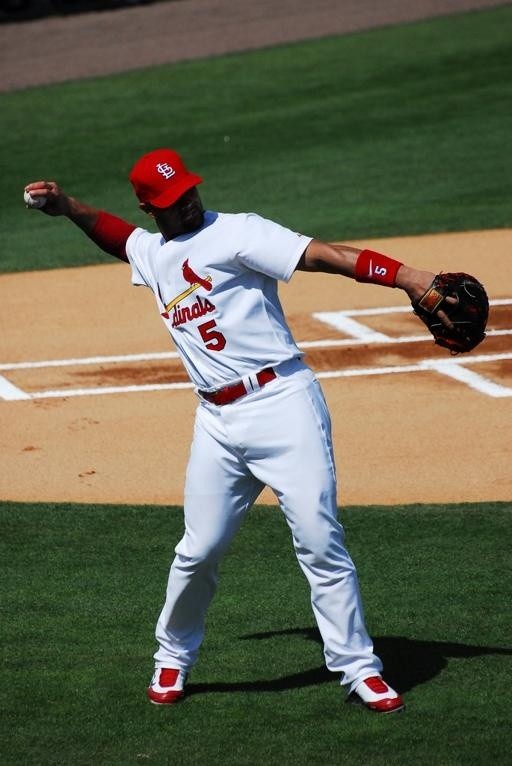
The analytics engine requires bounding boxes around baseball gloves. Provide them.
[412,272,489,352]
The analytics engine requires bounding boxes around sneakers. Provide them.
[146,668,188,705]
[354,677,405,715]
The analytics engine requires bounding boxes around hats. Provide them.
[129,149,203,209]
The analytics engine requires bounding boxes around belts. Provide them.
[198,367,276,406]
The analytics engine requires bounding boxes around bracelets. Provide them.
[355,248,404,289]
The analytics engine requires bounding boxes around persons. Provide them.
[23,144,461,716]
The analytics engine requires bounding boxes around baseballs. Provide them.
[24,190,47,209]
